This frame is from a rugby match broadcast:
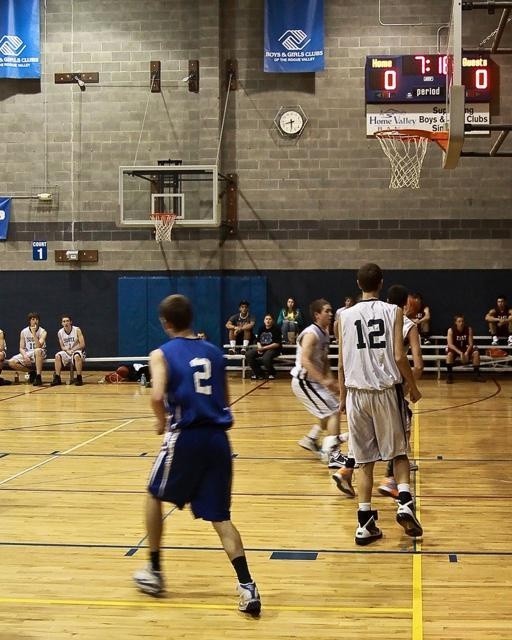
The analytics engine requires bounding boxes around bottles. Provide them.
[24,373,30,385]
[14,372,19,385]
[141,374,147,386]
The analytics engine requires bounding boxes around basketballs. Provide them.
[117,366,129,378]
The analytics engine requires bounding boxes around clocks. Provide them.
[273,105,308,136]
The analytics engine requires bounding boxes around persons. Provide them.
[225,300,256,354]
[198,331,208,340]
[247,313,282,380]
[8,313,48,386]
[290,264,431,545]
[49,314,86,386]
[445,313,486,383]
[132,294,262,618]
[0,330,7,374]
[485,297,512,347]
[277,298,304,344]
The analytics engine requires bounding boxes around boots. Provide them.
[28,371,36,383]
[491,335,499,345]
[508,336,512,346]
[51,374,61,385]
[33,374,42,386]
[76,375,82,385]
[473,373,485,382]
[355,511,382,545]
[395,492,422,536]
[447,372,453,384]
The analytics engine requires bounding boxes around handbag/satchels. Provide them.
[486,348,508,357]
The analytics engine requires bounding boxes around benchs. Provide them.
[224,335,512,379]
[4,354,247,379]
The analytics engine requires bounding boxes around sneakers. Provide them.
[424,338,431,344]
[409,462,418,471]
[328,451,359,468]
[268,374,275,379]
[298,436,320,451]
[241,349,246,354]
[333,466,355,496]
[133,570,163,594]
[229,347,235,354]
[281,340,286,343]
[321,436,337,462]
[237,581,261,611]
[251,375,263,379]
[377,477,414,499]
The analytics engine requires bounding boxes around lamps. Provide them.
[75,76,84,87]
[183,74,195,81]
